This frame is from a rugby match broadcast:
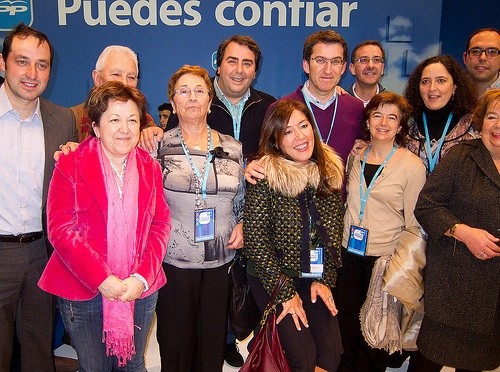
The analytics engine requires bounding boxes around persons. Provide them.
[463,27,500,97]
[262,30,368,203]
[350,55,482,174]
[140,35,276,367]
[146,64,244,372]
[37,80,171,372]
[407,87,500,372]
[242,99,344,372]
[69,45,156,144]
[157,102,173,129]
[334,91,427,372]
[0,23,82,372]
[335,40,387,108]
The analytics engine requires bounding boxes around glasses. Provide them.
[173,85,211,98]
[354,57,383,65]
[467,47,500,58]
[308,56,345,66]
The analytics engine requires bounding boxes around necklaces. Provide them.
[178,127,210,209]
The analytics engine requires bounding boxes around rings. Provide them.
[59,145,62,149]
[329,297,333,301]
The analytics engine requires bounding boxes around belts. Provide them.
[0,232,43,243]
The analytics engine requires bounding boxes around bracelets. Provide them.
[130,273,148,292]
[450,223,460,237]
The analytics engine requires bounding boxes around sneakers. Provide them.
[225,343,244,368]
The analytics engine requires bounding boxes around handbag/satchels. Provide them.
[238,275,291,372]
